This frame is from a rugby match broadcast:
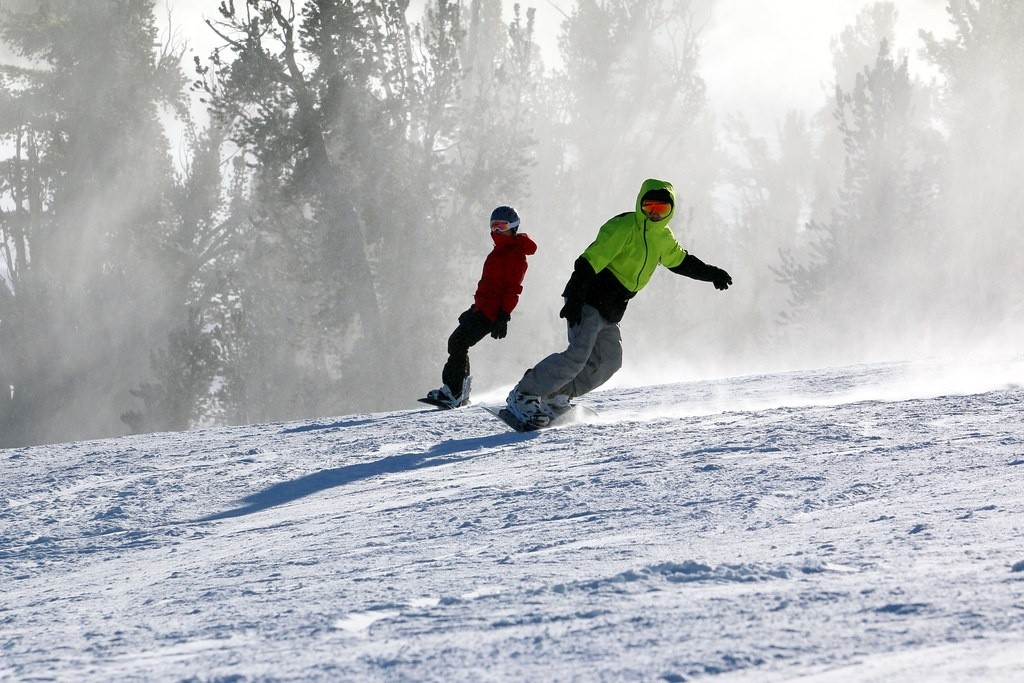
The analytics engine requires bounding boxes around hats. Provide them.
[643,188,674,206]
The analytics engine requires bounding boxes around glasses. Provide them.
[642,201,671,218]
[490,220,510,232]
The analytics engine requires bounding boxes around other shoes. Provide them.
[428,386,462,409]
[543,395,576,422]
[507,389,551,429]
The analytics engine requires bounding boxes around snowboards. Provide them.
[417,397,472,409]
[479,402,598,434]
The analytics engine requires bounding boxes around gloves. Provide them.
[457,304,474,324]
[559,289,583,328]
[490,310,511,338]
[708,265,733,290]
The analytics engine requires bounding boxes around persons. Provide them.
[506,178,732,429]
[425,206,530,406]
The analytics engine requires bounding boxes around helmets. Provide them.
[488,206,519,235]
